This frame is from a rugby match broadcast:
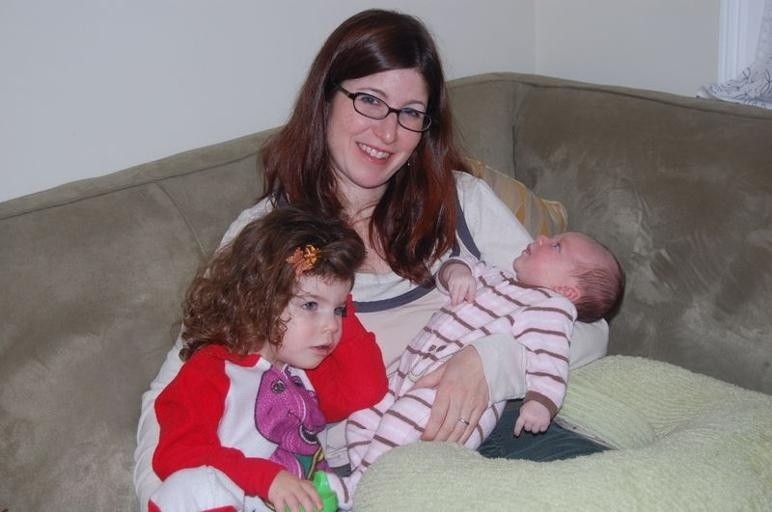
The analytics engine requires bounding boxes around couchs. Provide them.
[0,73,770,511]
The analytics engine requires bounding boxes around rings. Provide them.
[458,417,471,427]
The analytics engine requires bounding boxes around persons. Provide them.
[132,9,611,512]
[154,209,387,512]
[327,232,625,510]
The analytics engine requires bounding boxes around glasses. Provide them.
[337,86,432,132]
